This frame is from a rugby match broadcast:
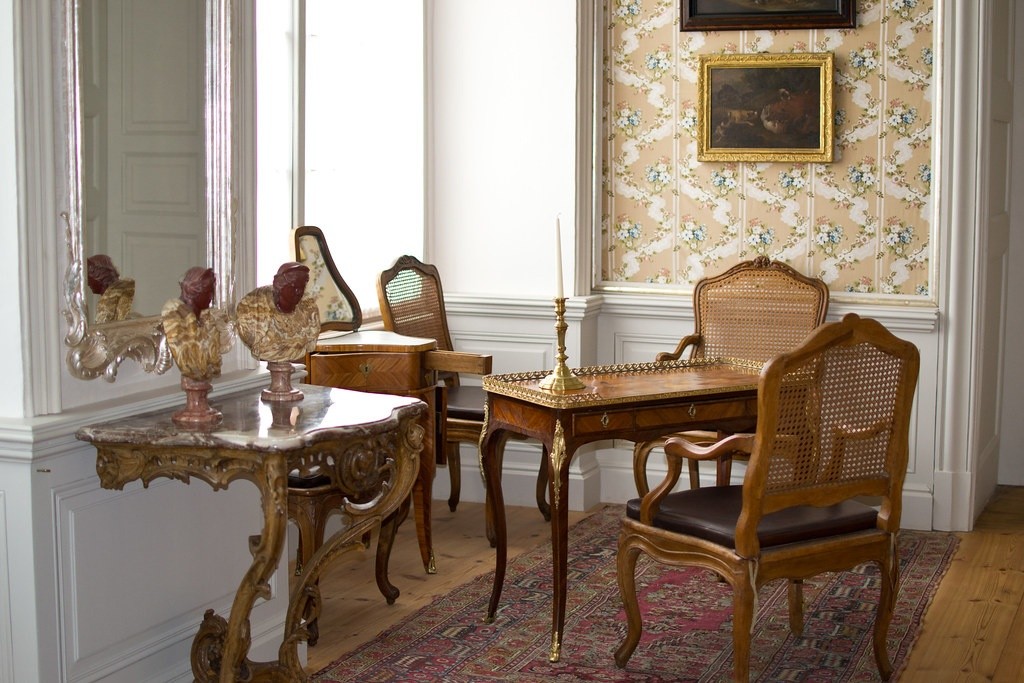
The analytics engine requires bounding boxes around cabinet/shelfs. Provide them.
[300,329,493,574]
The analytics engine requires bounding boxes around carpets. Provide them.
[305,502,962,683]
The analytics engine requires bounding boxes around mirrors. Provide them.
[61,0,239,382]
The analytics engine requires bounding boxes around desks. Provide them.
[481,355,817,663]
[74,381,430,683]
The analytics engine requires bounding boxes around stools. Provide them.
[287,476,400,646]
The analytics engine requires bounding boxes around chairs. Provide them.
[632,254,829,584]
[375,255,552,549]
[614,313,920,683]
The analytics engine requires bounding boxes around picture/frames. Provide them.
[680,0,857,33]
[697,51,835,163]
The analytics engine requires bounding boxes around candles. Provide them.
[555,216,564,299]
